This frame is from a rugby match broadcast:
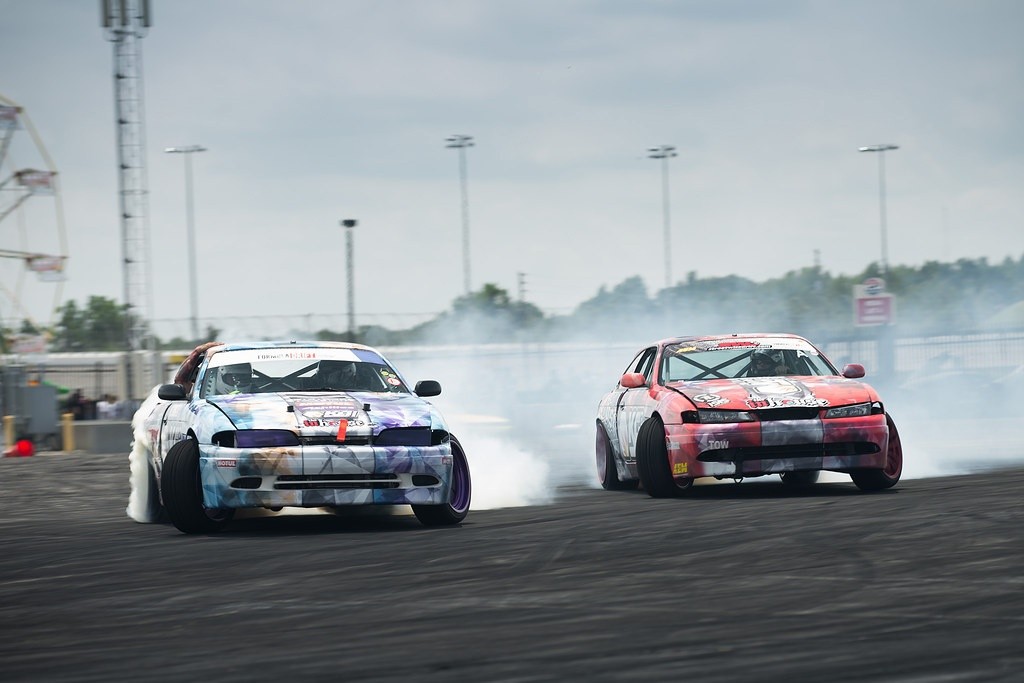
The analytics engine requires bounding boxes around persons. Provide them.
[68,387,122,421]
[175,340,255,399]
[751,349,793,376]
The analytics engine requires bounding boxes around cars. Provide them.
[595,331,903,489]
[126,339,471,535]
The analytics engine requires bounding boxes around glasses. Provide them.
[755,358,773,365]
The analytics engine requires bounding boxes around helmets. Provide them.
[753,349,784,362]
[319,360,357,377]
[216,362,253,394]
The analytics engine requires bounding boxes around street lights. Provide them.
[440,135,483,339]
[642,145,682,342]
[162,144,209,342]
[859,140,900,335]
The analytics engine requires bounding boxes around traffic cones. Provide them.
[2,438,35,458]
